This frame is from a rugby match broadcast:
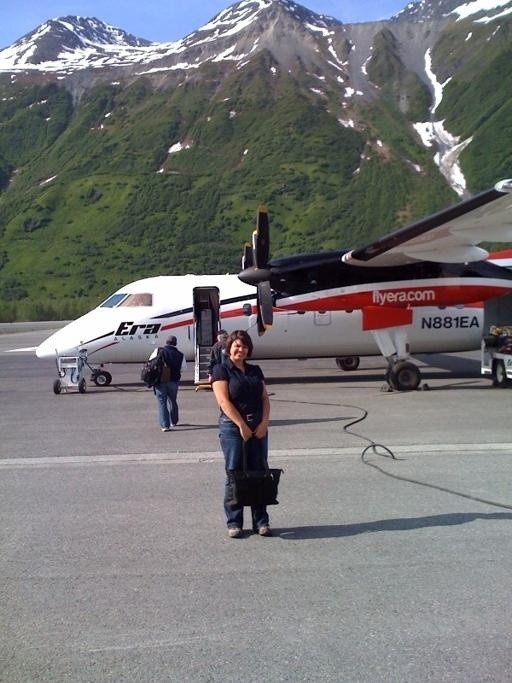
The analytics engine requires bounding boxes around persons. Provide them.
[210,327,275,538]
[148,333,186,431]
[206,329,231,383]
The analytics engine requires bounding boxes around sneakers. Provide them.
[257,527,271,536]
[171,423,175,427]
[228,528,242,537]
[160,426,169,432]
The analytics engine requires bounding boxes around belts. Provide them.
[243,414,258,421]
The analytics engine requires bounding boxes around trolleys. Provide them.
[52,348,86,393]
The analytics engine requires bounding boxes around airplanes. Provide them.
[35,177,511,391]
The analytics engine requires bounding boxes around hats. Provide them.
[166,335,177,343]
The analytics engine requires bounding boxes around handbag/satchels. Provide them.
[141,358,164,387]
[229,469,282,506]
